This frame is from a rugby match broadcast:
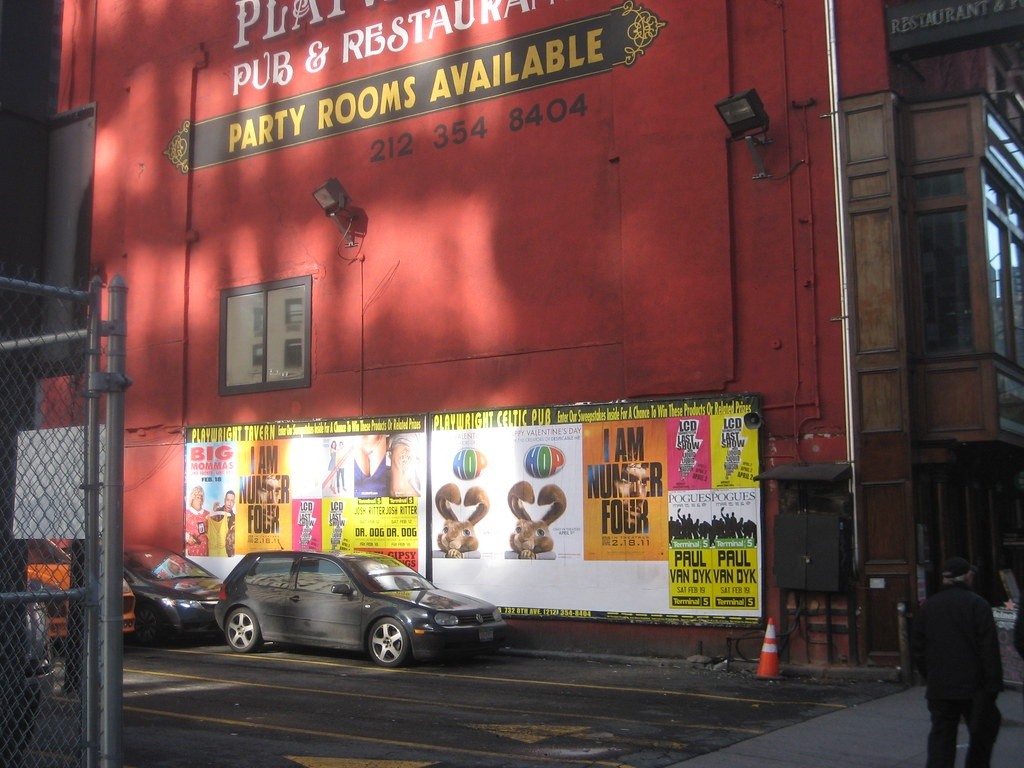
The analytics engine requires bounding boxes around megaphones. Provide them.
[743,411,765,431]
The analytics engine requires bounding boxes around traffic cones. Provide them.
[753,616,785,681]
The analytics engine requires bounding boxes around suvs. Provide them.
[8,536,137,638]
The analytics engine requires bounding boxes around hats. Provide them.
[939,557,969,578]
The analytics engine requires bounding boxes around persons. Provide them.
[184,485,212,558]
[213,490,236,558]
[329,431,423,500]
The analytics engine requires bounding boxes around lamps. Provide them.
[312,175,347,215]
[717,92,770,144]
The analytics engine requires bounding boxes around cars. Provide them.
[216,549,507,664]
[60,539,229,646]
